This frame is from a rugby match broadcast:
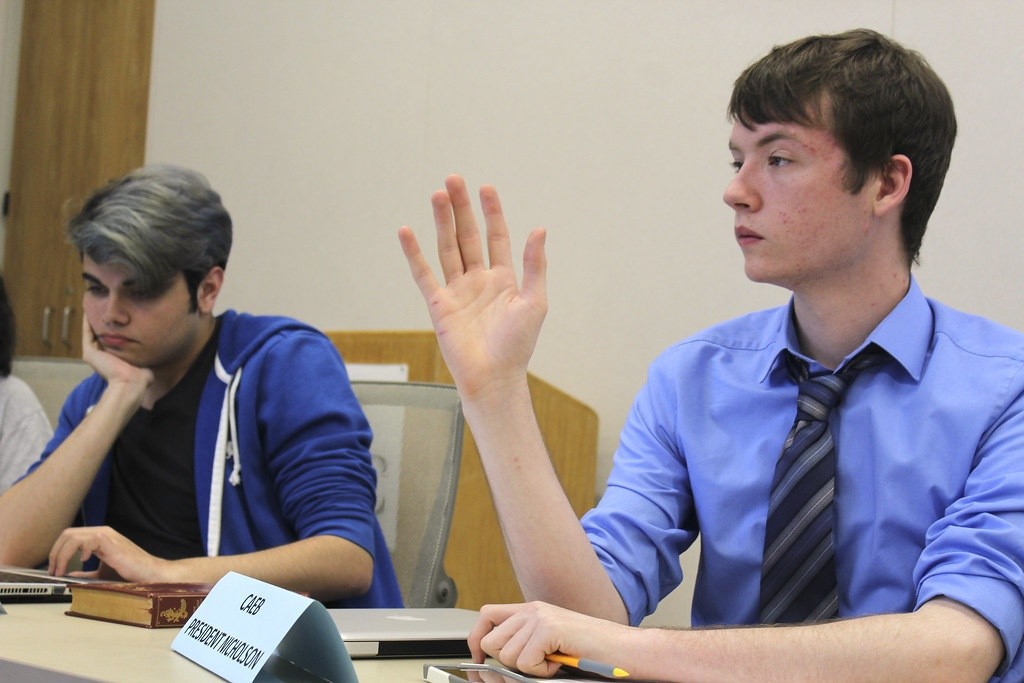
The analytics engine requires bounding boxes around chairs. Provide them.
[350,381,465,607]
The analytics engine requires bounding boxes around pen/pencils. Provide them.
[544,652,630,678]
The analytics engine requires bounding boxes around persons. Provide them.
[397,29,1024,682]
[0,278,54,497]
[0,161,403,609]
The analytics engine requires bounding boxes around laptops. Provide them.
[0,569,89,597]
[327,607,491,656]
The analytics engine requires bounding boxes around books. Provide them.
[66,580,210,629]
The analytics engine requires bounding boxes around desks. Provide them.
[0,599,572,683]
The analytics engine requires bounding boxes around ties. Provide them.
[759,344,890,622]
